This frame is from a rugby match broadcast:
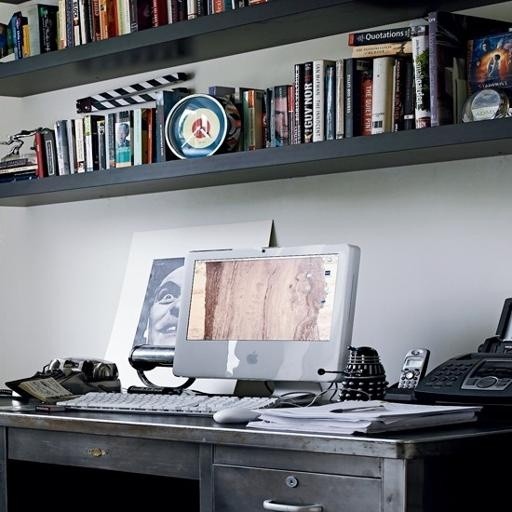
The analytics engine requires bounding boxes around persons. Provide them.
[146,264,183,348]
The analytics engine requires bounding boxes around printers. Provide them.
[414,297,512,409]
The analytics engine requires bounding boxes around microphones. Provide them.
[318,368,353,378]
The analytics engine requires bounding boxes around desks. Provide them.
[0,395,512,512]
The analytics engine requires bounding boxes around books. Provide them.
[0,0,512,182]
[17,376,85,404]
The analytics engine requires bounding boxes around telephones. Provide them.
[384,348,429,401]
[416,337,512,406]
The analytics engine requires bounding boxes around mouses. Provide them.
[213,406,261,423]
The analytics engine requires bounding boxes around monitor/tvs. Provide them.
[172,243,361,405]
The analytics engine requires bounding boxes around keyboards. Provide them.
[55,389,281,418]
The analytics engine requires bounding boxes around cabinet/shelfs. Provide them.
[1,0,512,207]
